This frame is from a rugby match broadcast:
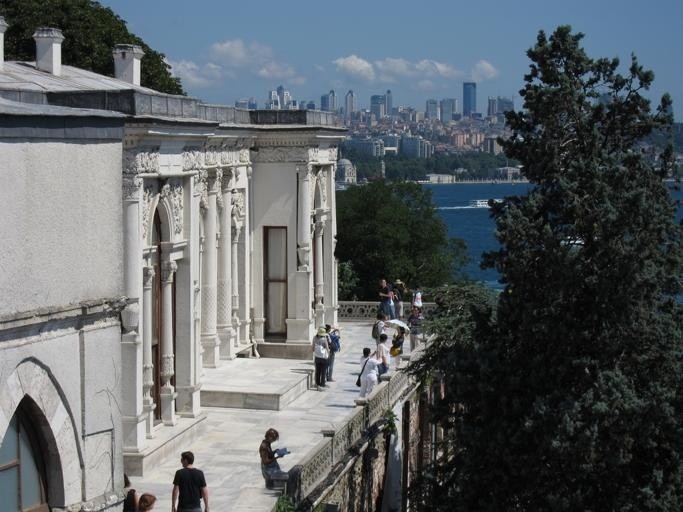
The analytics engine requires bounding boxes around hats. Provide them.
[317,328,326,337]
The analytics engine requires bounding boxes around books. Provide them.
[276,448,291,456]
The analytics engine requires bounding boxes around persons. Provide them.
[138,494,156,511]
[377,278,424,319]
[375,334,390,381]
[373,312,387,347]
[171,451,209,512]
[359,348,383,397]
[259,428,290,489]
[312,322,340,387]
[407,305,421,353]
[123,471,138,511]
[391,326,405,370]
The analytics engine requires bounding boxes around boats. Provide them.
[469,199,509,209]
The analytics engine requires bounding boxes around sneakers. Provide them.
[317,377,336,391]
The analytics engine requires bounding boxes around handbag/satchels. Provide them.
[390,346,401,356]
[356,378,361,387]
[314,343,330,359]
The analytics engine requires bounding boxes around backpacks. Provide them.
[372,320,381,339]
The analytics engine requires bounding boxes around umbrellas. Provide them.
[385,318,410,337]
